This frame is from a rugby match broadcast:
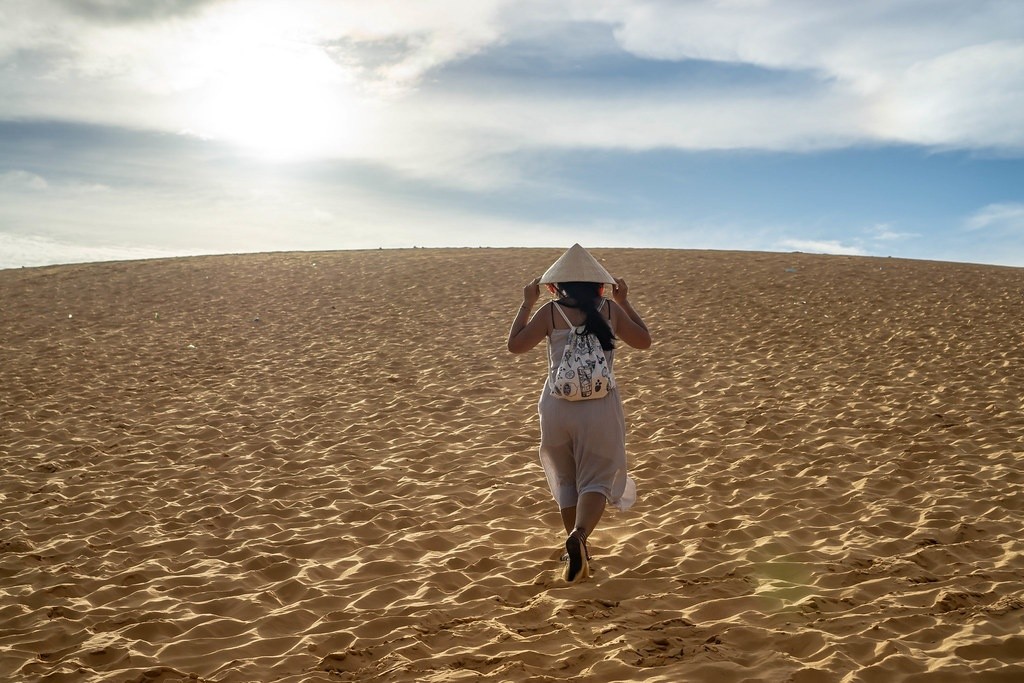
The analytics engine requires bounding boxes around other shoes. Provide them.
[561,531,592,581]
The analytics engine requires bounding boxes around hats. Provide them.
[534,244,619,287]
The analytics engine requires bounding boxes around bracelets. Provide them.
[521,305,532,314]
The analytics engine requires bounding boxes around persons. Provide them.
[508,244,651,584]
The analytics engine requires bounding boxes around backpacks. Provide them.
[548,297,618,401]
